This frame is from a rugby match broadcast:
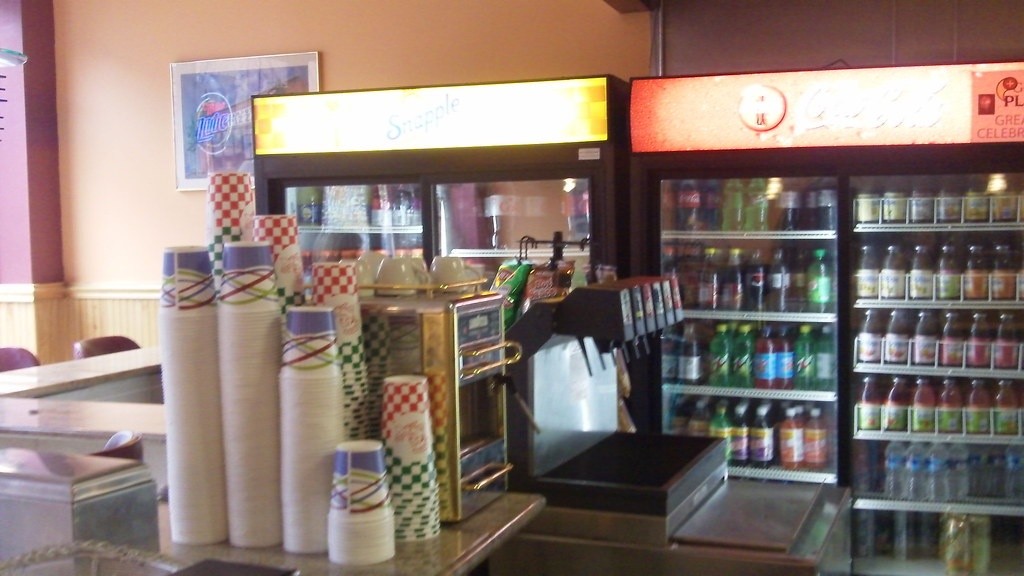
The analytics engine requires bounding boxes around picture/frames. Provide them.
[170,52,320,191]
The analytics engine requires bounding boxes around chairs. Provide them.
[0,347,40,373]
[73,335,142,361]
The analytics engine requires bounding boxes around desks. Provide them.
[0,339,169,489]
[0,432,547,576]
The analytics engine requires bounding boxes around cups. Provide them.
[312,262,371,442]
[327,441,396,563]
[209,173,254,296]
[279,307,344,554]
[218,242,282,547]
[253,213,308,307]
[382,375,441,540]
[338,251,483,296]
[160,247,228,545]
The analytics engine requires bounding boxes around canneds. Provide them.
[944,513,990,575]
[857,191,1024,222]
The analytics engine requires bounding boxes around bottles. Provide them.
[410,184,422,226]
[370,184,392,225]
[853,243,1023,503]
[299,187,323,224]
[392,184,409,226]
[567,180,591,242]
[661,176,835,468]
